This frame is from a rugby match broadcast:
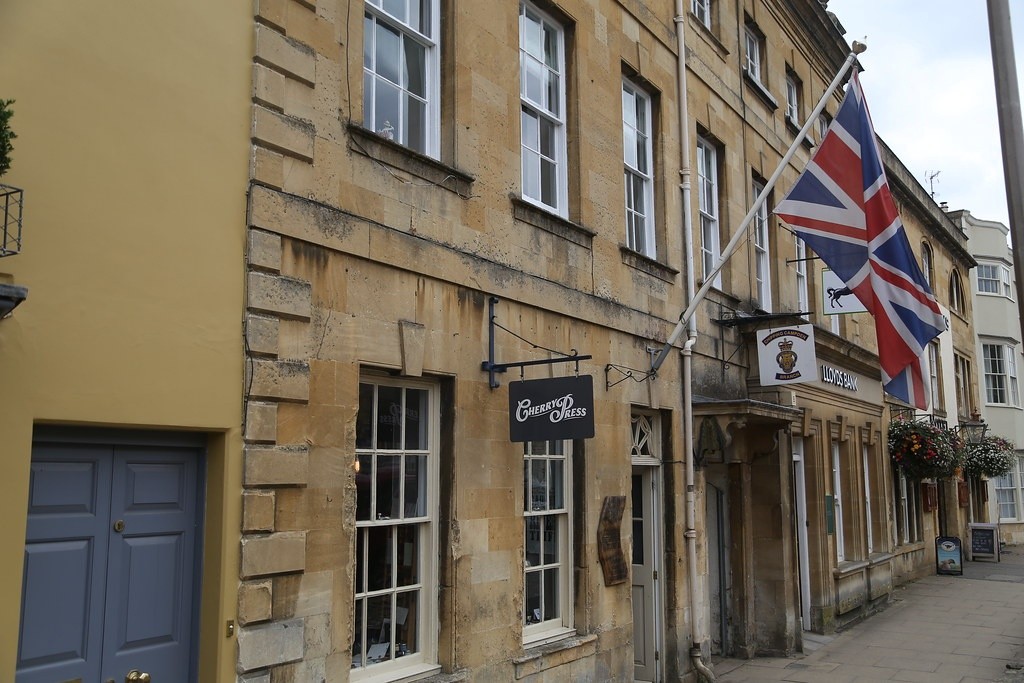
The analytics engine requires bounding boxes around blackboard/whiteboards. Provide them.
[968,524,998,557]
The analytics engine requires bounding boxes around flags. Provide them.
[772,66,951,411]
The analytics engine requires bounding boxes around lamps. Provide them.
[953,406,992,447]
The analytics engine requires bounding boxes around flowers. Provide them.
[890,417,953,481]
[944,427,971,470]
[963,435,1017,478]
